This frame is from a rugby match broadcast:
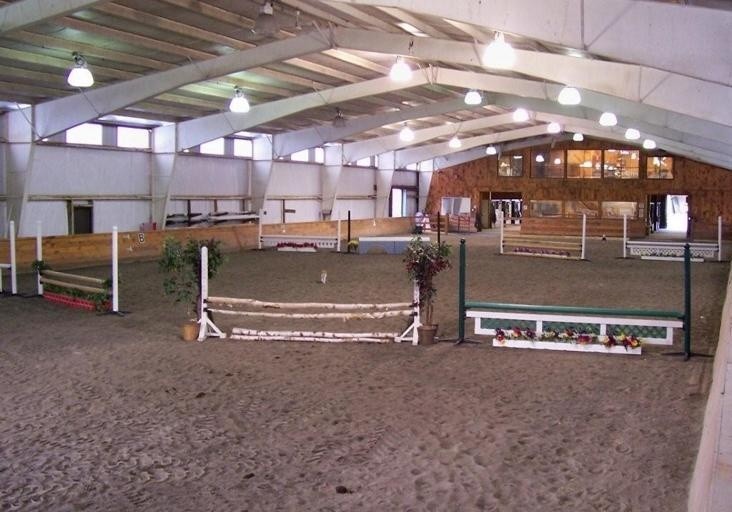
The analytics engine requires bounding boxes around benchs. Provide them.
[357,236,430,254]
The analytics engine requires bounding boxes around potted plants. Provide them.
[404,233,453,345]
[43,281,112,311]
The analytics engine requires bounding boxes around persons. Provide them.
[474,205,482,232]
[491,202,496,229]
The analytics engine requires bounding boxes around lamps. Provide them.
[228,87,250,114]
[64,56,95,89]
[332,107,347,129]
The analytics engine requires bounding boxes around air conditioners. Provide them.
[156,232,228,342]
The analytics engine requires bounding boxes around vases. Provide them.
[277,247,316,252]
[350,248,356,253]
[492,339,643,356]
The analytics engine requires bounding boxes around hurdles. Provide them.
[257,209,341,254]
[498,212,588,261]
[436,239,715,363]
[197,247,427,345]
[34,221,131,317]
[622,214,722,263]
[347,211,440,255]
[0,220,19,294]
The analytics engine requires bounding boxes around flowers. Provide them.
[347,240,358,250]
[494,325,645,353]
[276,241,318,250]
